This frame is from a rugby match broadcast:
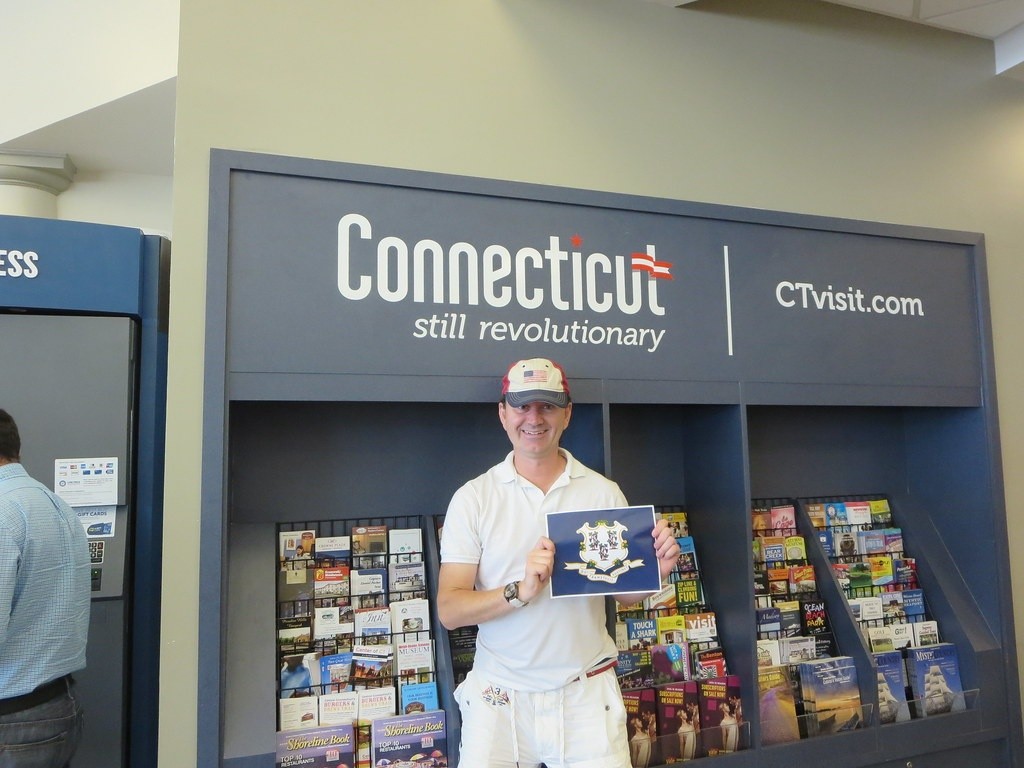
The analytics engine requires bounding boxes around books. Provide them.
[656,680,703,764]
[274,526,448,768]
[615,512,745,688]
[621,687,659,768]
[803,499,967,726]
[448,625,479,685]
[698,674,745,757]
[752,505,864,746]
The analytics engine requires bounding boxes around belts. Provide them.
[1,671,75,717]
[575,655,618,681]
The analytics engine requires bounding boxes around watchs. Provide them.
[503,581,529,608]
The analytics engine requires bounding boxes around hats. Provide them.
[501,357,570,409]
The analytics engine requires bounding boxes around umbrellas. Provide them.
[377,758,391,765]
[410,753,427,760]
[431,750,443,757]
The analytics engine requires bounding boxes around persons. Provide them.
[717,694,743,752]
[437,357,681,768]
[676,697,701,761]
[294,545,310,559]
[639,637,644,644]
[280,655,314,698]
[629,707,658,768]
[0,409,92,768]
